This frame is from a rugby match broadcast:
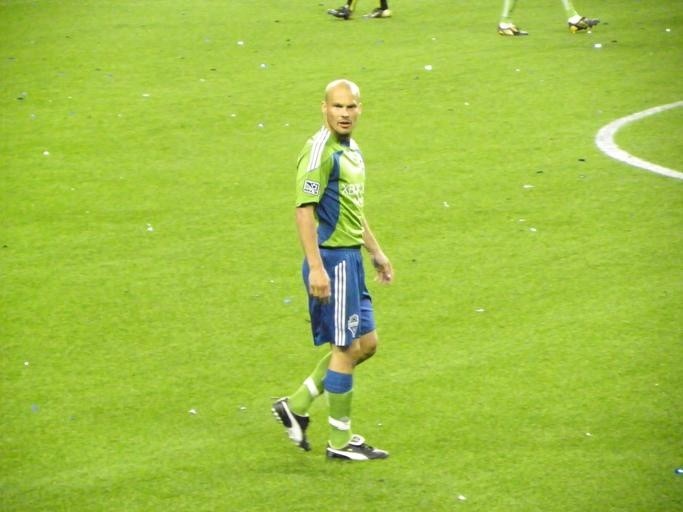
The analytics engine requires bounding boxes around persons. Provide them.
[324,0,392,21]
[268,78,395,462]
[496,1,602,37]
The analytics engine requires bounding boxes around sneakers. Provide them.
[567,16,600,33]
[362,7,391,20]
[327,6,351,19]
[271,397,309,452]
[325,434,390,461]
[497,23,528,36]
